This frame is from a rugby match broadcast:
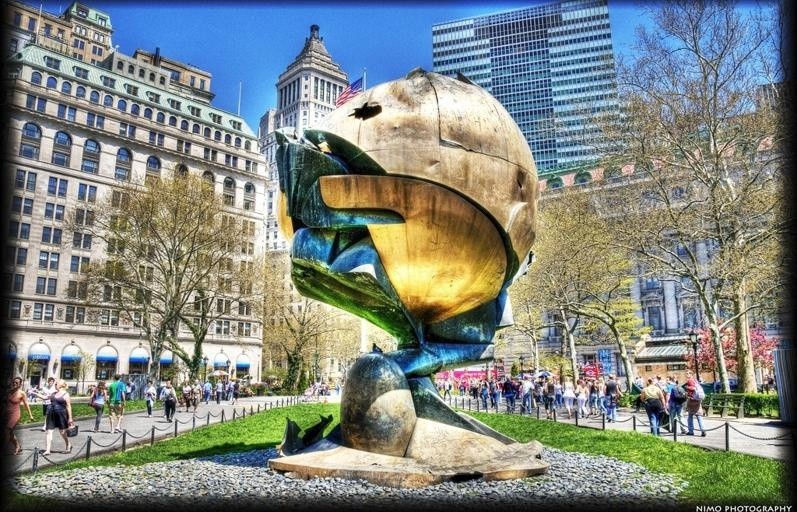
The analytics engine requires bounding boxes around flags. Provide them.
[335,77,363,109]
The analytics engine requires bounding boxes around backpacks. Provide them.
[668,383,687,403]
[687,378,705,401]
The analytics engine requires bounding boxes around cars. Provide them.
[713,378,740,392]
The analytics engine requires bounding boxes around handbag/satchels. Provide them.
[611,394,620,403]
[168,389,176,404]
[67,422,78,437]
[91,388,96,407]
[646,398,664,415]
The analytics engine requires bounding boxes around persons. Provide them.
[433,359,707,437]
[768,378,774,391]
[5,374,254,456]
[303,381,340,402]
